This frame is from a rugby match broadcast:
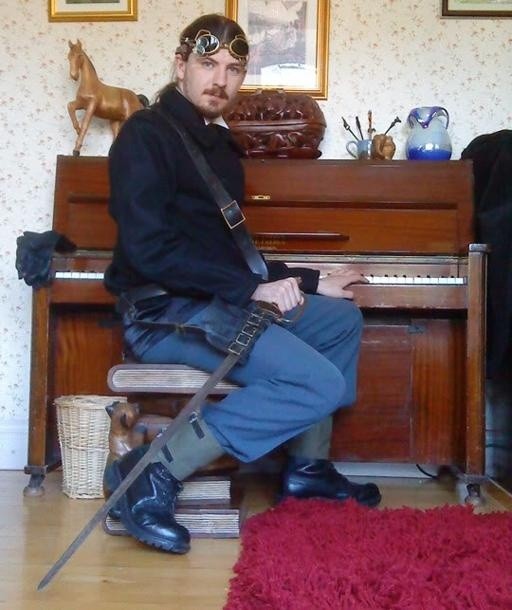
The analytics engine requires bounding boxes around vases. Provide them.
[405,106,454,161]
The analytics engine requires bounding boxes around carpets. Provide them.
[218,491,511,610]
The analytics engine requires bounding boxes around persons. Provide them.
[104,13,382,552]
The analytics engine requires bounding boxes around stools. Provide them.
[103,357,244,545]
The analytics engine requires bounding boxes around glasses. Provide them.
[179,29,250,65]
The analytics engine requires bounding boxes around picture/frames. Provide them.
[439,0,512,22]
[44,0,143,25]
[222,0,332,101]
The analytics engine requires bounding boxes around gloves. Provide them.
[15,231,78,289]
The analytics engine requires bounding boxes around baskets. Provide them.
[53,395,128,499]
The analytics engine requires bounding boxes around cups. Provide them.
[346,139,373,160]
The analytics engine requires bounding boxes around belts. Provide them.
[117,284,168,310]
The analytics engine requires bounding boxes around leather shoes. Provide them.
[104,444,190,555]
[276,457,381,507]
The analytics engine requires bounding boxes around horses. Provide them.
[67,38,149,157]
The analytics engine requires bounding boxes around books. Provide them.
[173,468,235,506]
[102,484,249,539]
[107,349,246,397]
